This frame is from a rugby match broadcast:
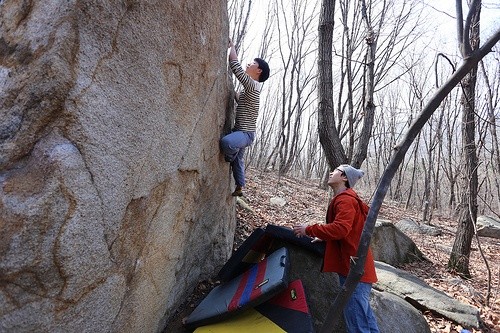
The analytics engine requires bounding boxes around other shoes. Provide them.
[232,189,243,196]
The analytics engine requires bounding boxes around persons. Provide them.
[220,39,269,197]
[292,162,381,333]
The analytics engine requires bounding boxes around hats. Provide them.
[340,163,364,188]
